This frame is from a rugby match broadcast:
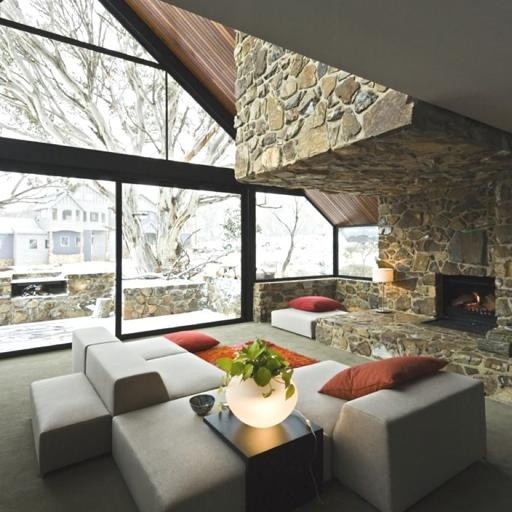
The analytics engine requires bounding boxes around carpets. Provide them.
[193,339,318,373]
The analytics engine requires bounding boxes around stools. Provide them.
[29,373,115,474]
[270,307,349,340]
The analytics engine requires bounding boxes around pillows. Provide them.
[319,354,449,398]
[287,294,344,311]
[161,329,222,352]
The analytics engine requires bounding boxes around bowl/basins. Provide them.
[189,394,215,416]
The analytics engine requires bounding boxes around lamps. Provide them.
[372,268,393,313]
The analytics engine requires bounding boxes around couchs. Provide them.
[72,325,487,512]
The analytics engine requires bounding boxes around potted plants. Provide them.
[216,339,302,430]
